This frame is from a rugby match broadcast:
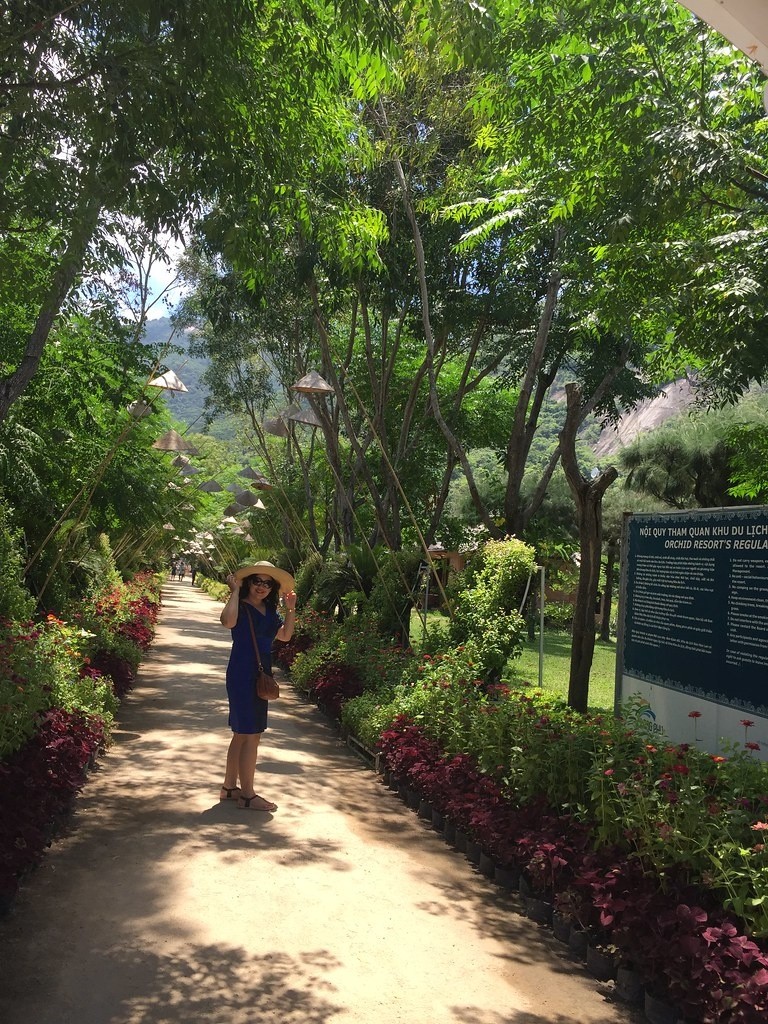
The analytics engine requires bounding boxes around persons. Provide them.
[219,560,297,810]
[171,561,197,585]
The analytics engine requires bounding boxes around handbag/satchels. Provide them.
[256,670,279,701]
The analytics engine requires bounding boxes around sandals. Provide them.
[219,786,241,800]
[236,792,275,811]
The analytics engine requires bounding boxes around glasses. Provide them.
[253,576,274,590]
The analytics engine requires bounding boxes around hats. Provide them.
[234,561,295,595]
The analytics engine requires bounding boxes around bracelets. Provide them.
[286,608,296,612]
